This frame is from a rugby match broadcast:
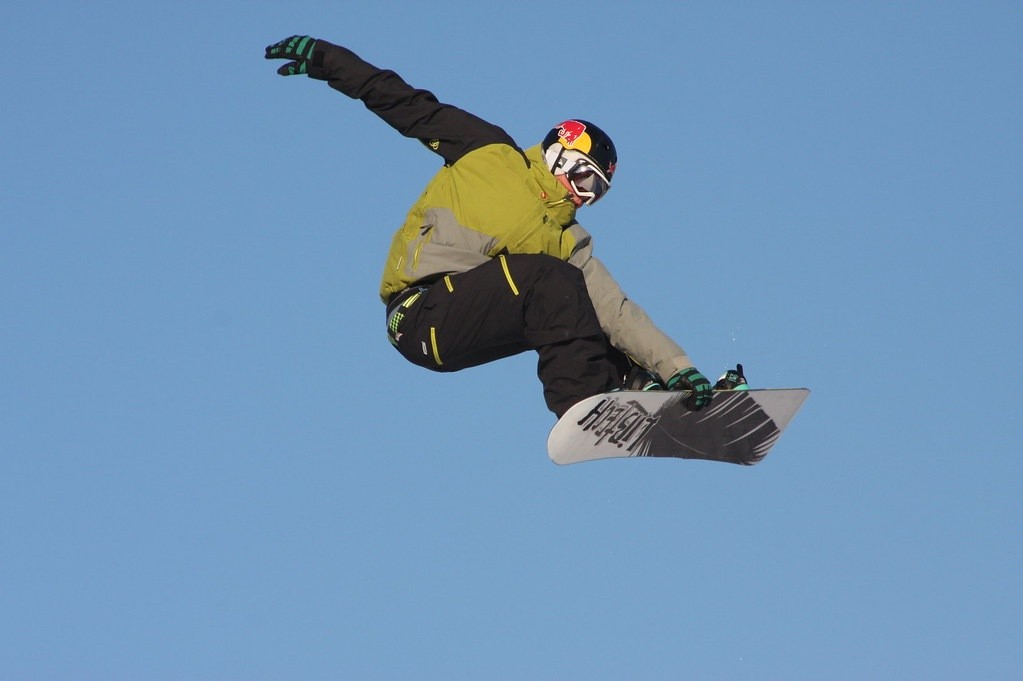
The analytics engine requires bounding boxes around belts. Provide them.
[387,289,425,347]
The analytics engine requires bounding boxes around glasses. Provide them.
[558,156,611,206]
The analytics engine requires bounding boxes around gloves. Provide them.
[665,366,713,411]
[264,35,316,76]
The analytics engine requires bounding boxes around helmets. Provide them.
[542,120,618,201]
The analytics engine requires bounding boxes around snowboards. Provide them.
[545,386,811,468]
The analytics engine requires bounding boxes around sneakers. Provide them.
[711,364,750,391]
[622,366,662,391]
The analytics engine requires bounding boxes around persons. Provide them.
[264,34,750,422]
